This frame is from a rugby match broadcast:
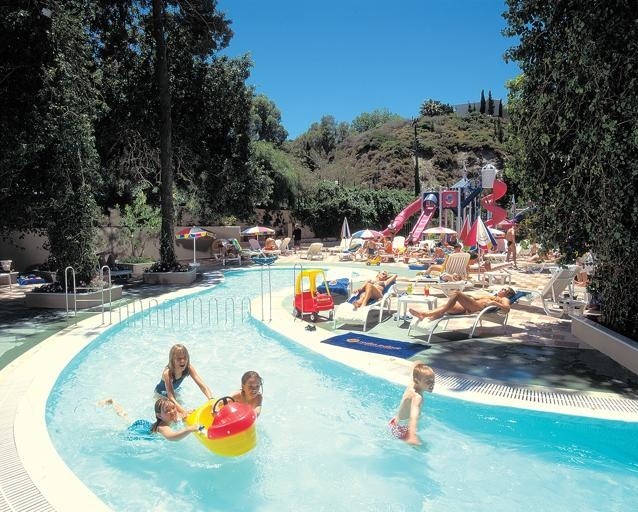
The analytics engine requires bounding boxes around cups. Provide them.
[407,286,412,295]
[424,288,430,298]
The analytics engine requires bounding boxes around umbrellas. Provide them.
[175,224,217,263]
[458,217,472,241]
[464,215,498,255]
[239,225,275,241]
[340,216,352,250]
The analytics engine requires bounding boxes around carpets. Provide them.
[319,333,433,362]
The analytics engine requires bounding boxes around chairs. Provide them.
[210,238,324,266]
[316,236,584,344]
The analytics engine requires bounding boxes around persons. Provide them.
[96,397,200,443]
[350,219,595,287]
[352,270,398,308]
[408,285,515,322]
[224,370,264,419]
[292,224,302,254]
[387,359,437,447]
[151,343,214,423]
[264,237,274,250]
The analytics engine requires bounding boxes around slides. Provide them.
[481,180,508,230]
[405,209,436,245]
[382,198,421,242]
